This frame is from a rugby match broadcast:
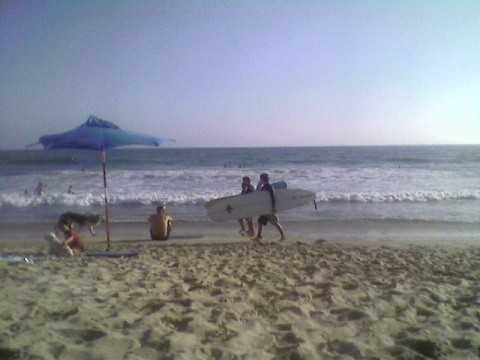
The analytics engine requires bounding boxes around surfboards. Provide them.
[207,190,319,220]
[205,181,288,207]
[3,252,140,262]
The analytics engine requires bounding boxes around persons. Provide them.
[250,173,286,242]
[67,184,75,195]
[239,177,254,236]
[34,181,45,195]
[149,206,173,240]
[60,219,84,255]
